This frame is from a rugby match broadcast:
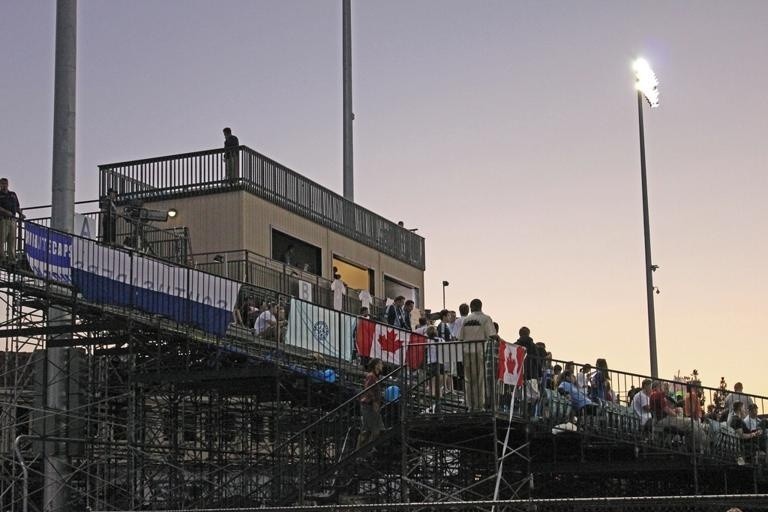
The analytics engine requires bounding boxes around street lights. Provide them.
[632,55,660,393]
[442,281,448,310]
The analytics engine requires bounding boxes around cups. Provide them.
[677,407,683,418]
[304,263,309,272]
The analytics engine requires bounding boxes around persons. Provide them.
[415,299,615,426]
[333,267,342,280]
[627,380,768,463]
[355,358,392,462]
[351,294,415,380]
[399,221,417,233]
[0,178,26,261]
[100,189,118,244]
[221,127,239,180]
[233,290,289,343]
[284,244,295,265]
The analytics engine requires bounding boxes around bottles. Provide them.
[546,368,550,379]
